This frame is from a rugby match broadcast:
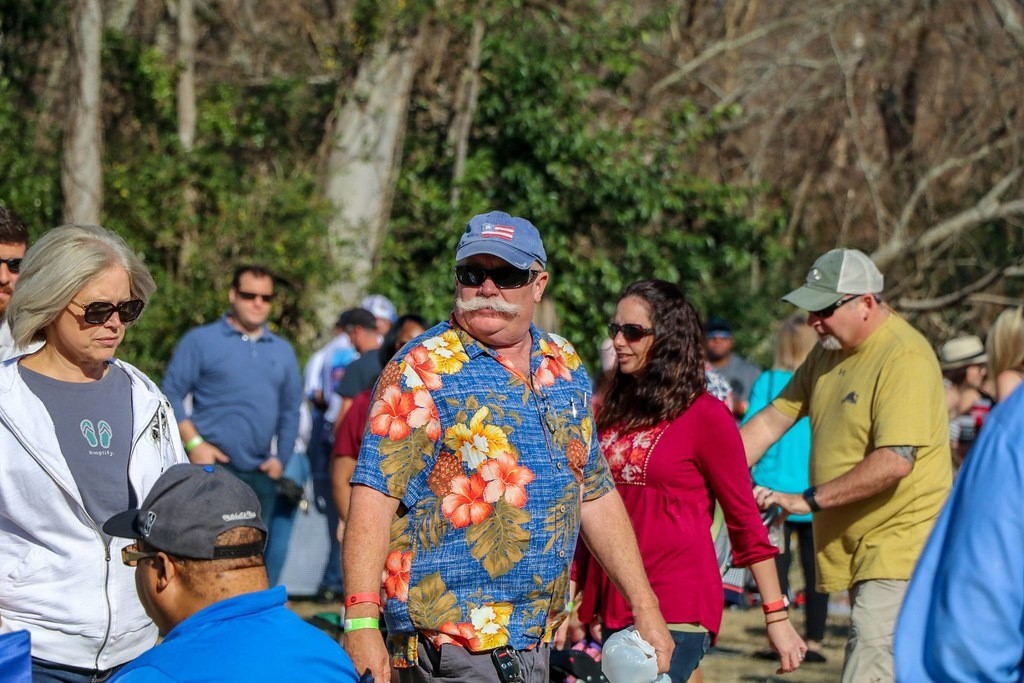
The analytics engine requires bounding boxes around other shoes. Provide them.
[753,649,827,662]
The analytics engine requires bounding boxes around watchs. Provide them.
[804,485,820,513]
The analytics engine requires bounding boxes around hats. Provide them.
[359,294,397,324]
[102,463,268,559]
[939,335,989,369]
[781,248,883,311]
[335,307,377,329]
[455,211,547,270]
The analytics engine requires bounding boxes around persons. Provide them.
[97,462,362,683]
[552,279,808,683]
[161,263,1024,683]
[336,210,675,683]
[0,219,188,683]
[0,220,48,362]
[736,247,953,683]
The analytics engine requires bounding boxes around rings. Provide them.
[798,653,804,658]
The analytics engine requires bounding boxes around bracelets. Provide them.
[761,594,790,613]
[766,617,789,625]
[345,593,381,609]
[344,617,380,634]
[185,434,204,453]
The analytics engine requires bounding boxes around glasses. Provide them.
[71,299,145,325]
[0,258,23,272]
[813,295,862,318]
[455,265,540,289]
[122,543,185,567]
[237,290,275,301]
[608,323,656,341]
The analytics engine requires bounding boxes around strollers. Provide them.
[549,483,777,683]
